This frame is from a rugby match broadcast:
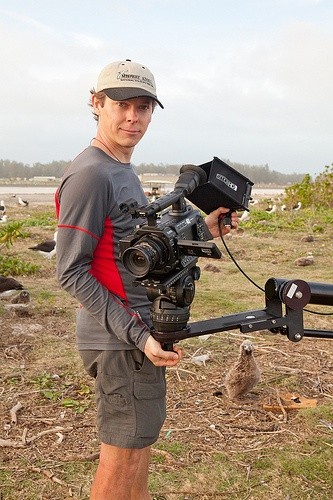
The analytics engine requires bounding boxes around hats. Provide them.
[97,59,164,110]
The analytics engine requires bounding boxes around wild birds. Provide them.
[224,340,261,402]
[293,252,315,266]
[27,232,57,259]
[0,194,29,223]
[0,275,24,294]
[249,198,301,214]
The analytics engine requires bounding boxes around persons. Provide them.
[54,59,240,500]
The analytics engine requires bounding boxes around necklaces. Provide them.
[94,137,122,163]
